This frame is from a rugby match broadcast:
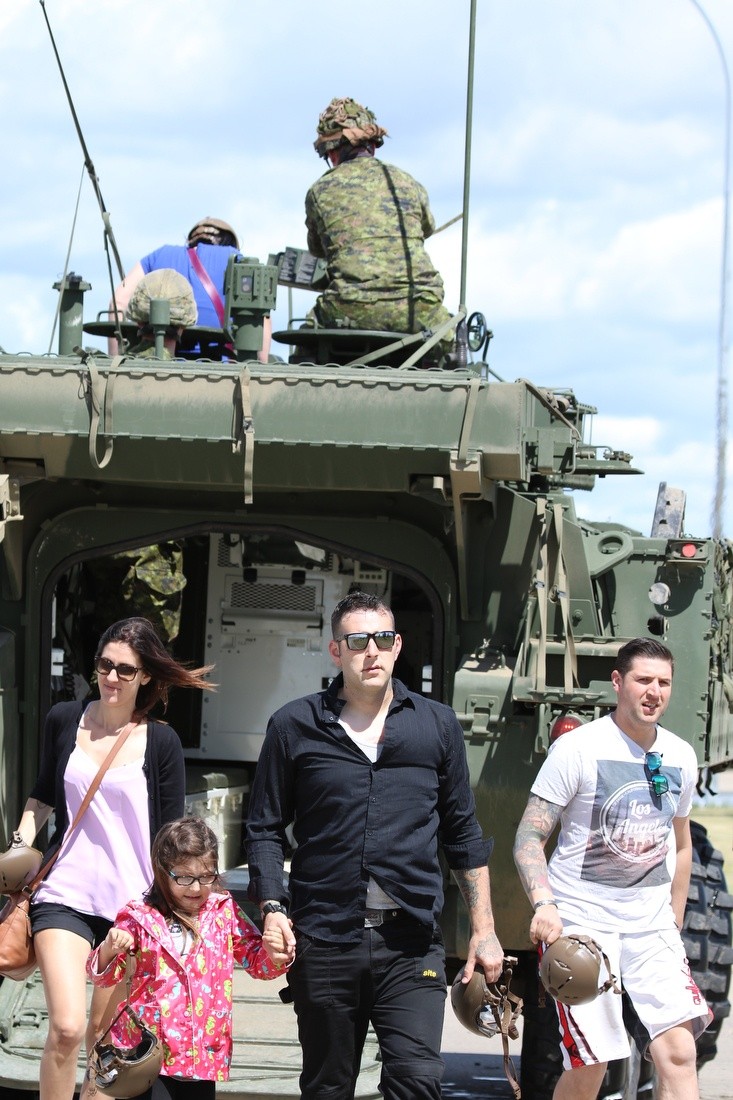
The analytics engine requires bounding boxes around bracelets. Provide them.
[261,902,288,922]
[533,900,558,914]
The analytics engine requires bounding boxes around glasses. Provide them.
[646,752,669,797]
[95,656,146,681]
[166,868,219,885]
[335,630,397,651]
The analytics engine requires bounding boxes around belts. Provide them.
[363,909,412,928]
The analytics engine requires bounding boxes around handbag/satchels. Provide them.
[0,890,38,981]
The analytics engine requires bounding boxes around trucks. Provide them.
[1,253,733,1100]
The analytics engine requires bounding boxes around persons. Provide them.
[512,638,714,1100]
[243,593,505,1100]
[109,215,271,364]
[17,617,220,1100]
[294,98,455,361]
[86,820,295,1100]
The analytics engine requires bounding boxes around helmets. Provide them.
[451,957,518,1039]
[538,933,602,1006]
[187,218,241,251]
[125,268,198,327]
[314,97,384,158]
[93,1025,163,1099]
[0,831,43,893]
